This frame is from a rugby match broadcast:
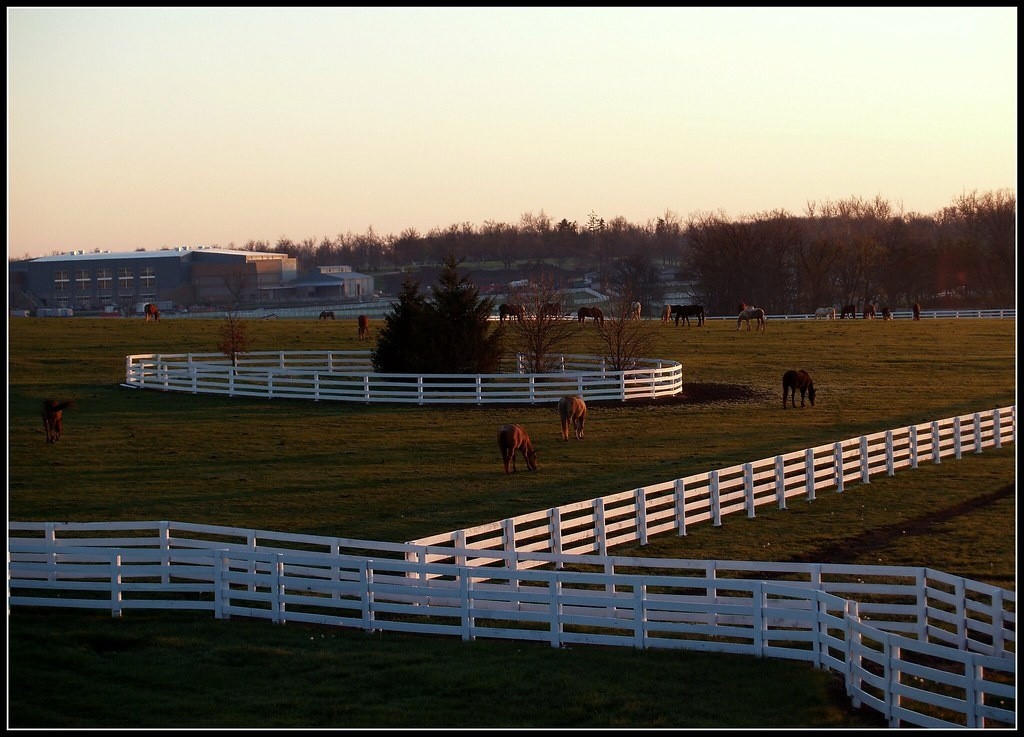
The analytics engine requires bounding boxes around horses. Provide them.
[913,303,921,321]
[862,304,875,320]
[814,307,835,320]
[557,394,588,443]
[144,304,161,322]
[41,398,73,444]
[499,303,525,323]
[782,370,817,409]
[736,304,766,331]
[539,303,561,321]
[578,307,605,328]
[839,304,856,319]
[358,315,372,341]
[625,301,642,321]
[319,310,336,320]
[498,423,538,475]
[882,308,893,321]
[662,304,705,328]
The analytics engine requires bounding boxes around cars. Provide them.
[461,279,534,295]
